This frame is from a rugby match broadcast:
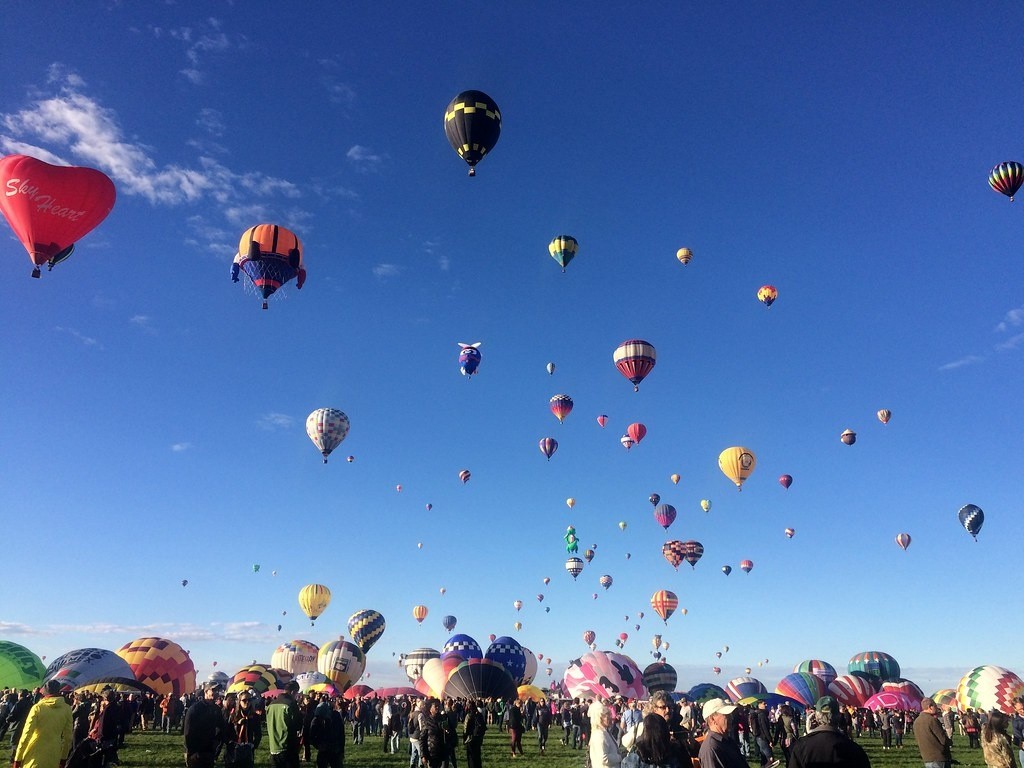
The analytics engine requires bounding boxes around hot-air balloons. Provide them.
[0,153,117,278]
[597,414,609,430]
[612,338,656,393]
[876,409,891,425]
[840,429,857,448]
[444,89,502,178]
[621,435,632,453]
[0,471,1024,718]
[306,407,354,465]
[626,423,646,446]
[718,447,757,491]
[457,341,483,379]
[539,437,558,464]
[757,285,779,310]
[548,393,574,425]
[548,235,579,273]
[229,223,307,310]
[989,161,1024,202]
[546,362,556,376]
[676,247,692,266]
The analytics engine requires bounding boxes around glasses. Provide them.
[240,698,253,702]
[656,703,675,709]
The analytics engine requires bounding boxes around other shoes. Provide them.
[577,744,582,750]
[521,754,526,757]
[895,745,905,750]
[511,753,517,757]
[560,738,568,746]
[883,745,891,750]
[572,743,576,749]
[539,748,548,756]
[353,738,363,746]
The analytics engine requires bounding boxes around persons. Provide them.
[0,681,1024,768]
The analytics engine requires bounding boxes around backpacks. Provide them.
[408,718,416,735]
[392,714,401,732]
[494,702,502,713]
[473,712,487,738]
[965,717,977,728]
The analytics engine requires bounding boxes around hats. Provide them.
[168,692,174,696]
[355,693,361,699]
[815,695,839,713]
[613,694,623,699]
[966,708,972,712]
[204,682,223,693]
[626,697,636,703]
[679,697,688,703]
[540,698,545,702]
[702,697,739,720]
